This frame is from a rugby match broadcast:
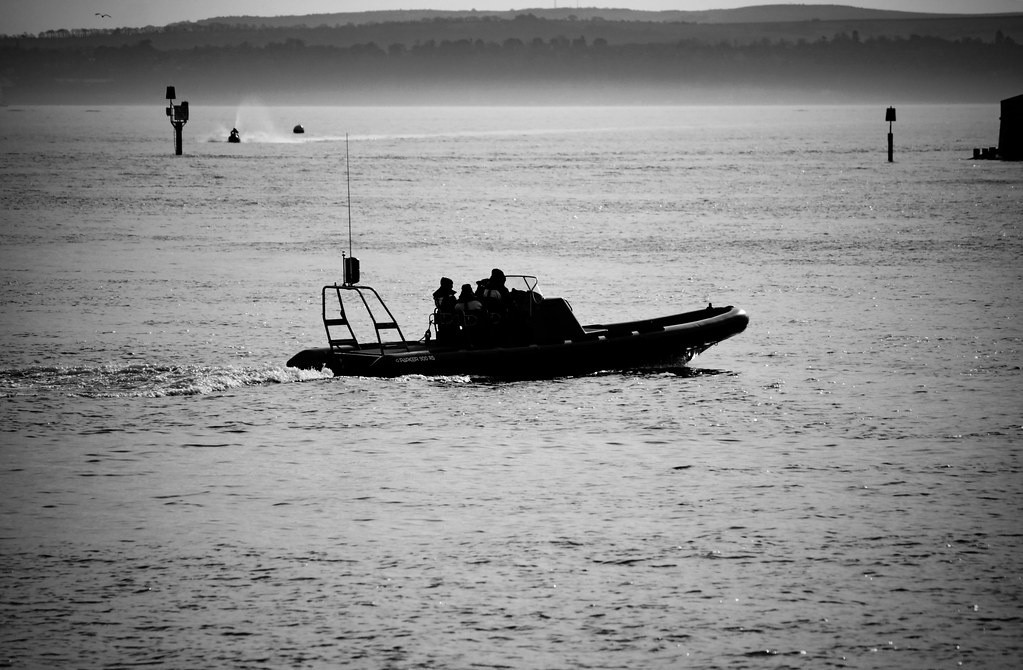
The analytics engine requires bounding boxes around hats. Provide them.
[459,284,474,299]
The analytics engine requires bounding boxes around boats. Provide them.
[283,132,751,385]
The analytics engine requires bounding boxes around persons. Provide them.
[431,277,457,311]
[459,284,474,298]
[231,128,239,133]
[481,268,510,297]
